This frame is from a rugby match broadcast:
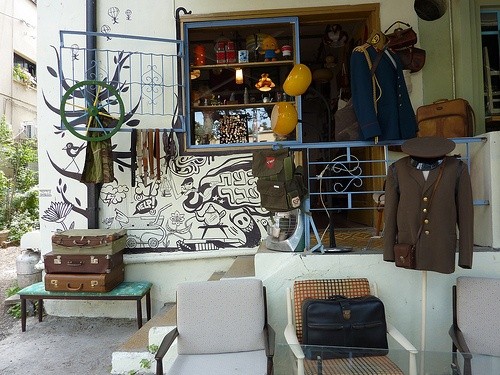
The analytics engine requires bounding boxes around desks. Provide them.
[16,281,153,333]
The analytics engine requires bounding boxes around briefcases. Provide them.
[43,229,127,293]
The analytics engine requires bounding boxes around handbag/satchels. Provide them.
[394,244,417,269]
[415,98,477,138]
[299,295,390,360]
[384,21,417,50]
[399,47,426,73]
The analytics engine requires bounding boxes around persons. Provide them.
[255,73,275,103]
[258,35,281,61]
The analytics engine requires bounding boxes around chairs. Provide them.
[155,278,275,375]
[283,277,418,375]
[449,276,500,375]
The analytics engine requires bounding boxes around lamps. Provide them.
[271,65,353,252]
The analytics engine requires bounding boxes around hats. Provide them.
[402,137,456,171]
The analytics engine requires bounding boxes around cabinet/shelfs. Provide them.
[179,2,386,230]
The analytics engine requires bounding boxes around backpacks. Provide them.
[251,148,309,212]
